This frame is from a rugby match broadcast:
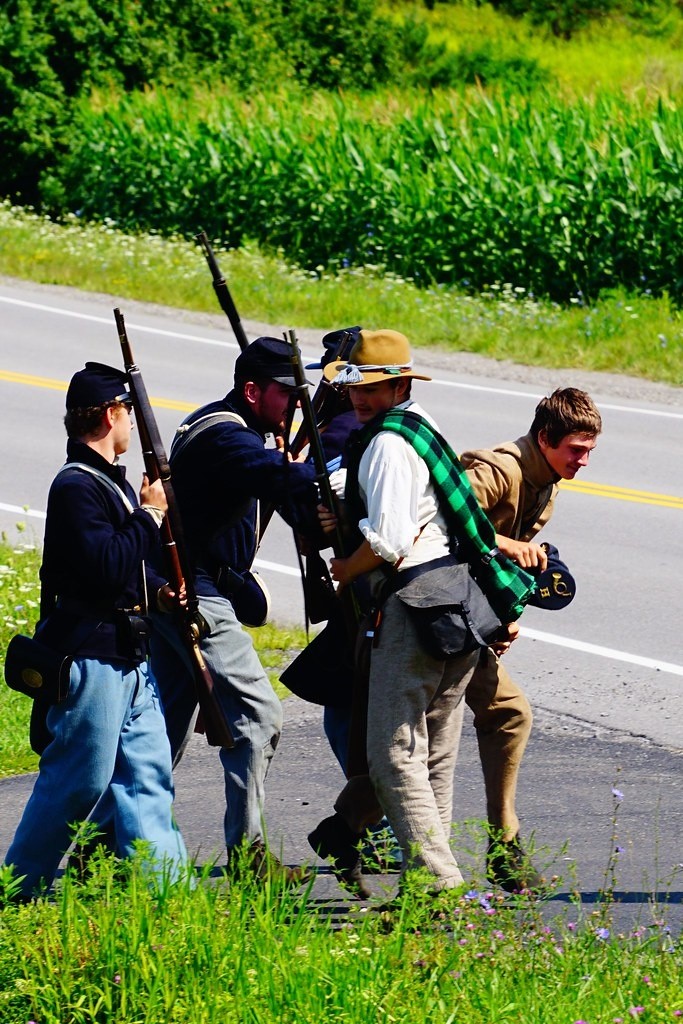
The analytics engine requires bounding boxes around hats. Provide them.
[303,325,362,369]
[233,336,315,388]
[65,362,133,408]
[324,329,432,386]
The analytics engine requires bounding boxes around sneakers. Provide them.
[308,813,372,900]
[486,833,547,895]
[227,841,313,897]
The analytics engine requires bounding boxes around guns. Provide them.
[284,328,358,556]
[111,307,241,748]
[261,330,357,540]
[194,228,340,625]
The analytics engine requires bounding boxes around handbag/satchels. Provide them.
[5,634,72,704]
[395,562,502,661]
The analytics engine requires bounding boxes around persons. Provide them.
[317,329,479,921]
[0,362,197,903]
[146,336,319,894]
[294,326,405,872]
[458,388,600,893]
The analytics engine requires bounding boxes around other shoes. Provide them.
[380,891,442,926]
